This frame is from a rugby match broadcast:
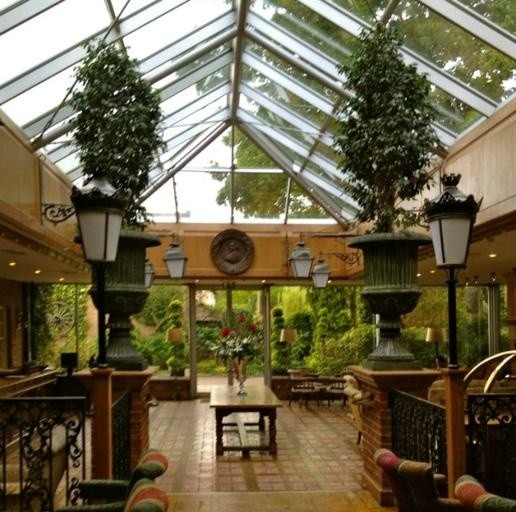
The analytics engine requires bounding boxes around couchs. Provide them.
[79,448,168,500]
[374,446,460,511]
[454,473,516,511]
[58,479,169,512]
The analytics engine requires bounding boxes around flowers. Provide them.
[210,308,265,365]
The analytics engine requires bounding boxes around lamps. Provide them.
[423,173,485,369]
[311,251,331,289]
[474,276,479,286]
[166,329,184,401]
[465,279,469,286]
[145,257,154,287]
[70,174,130,368]
[490,272,496,284]
[163,232,187,279]
[426,327,446,371]
[287,232,315,280]
[280,329,298,400]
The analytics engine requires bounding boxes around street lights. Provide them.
[67,173,133,483]
[418,172,484,500]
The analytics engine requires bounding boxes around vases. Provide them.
[234,356,251,395]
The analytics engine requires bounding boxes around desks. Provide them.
[209,385,284,459]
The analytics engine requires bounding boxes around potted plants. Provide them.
[329,21,447,372]
[66,37,167,371]
